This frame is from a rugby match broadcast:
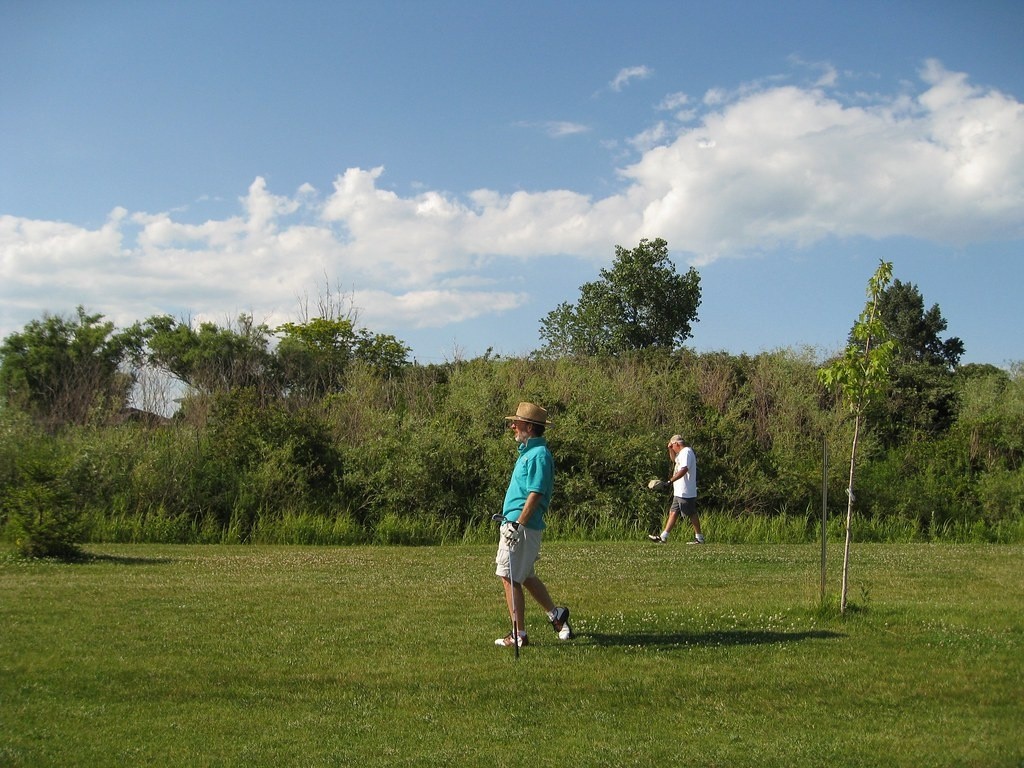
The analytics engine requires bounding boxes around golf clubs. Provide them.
[491,515,519,659]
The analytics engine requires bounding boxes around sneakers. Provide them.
[495,629,529,647]
[648,535,667,543]
[686,537,705,545]
[547,607,572,640]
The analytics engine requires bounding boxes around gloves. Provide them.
[661,481,672,490]
[502,521,527,553]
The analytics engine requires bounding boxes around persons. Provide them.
[649,435,705,545]
[494,401,571,647]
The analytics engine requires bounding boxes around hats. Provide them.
[669,435,684,445]
[504,402,555,427]
